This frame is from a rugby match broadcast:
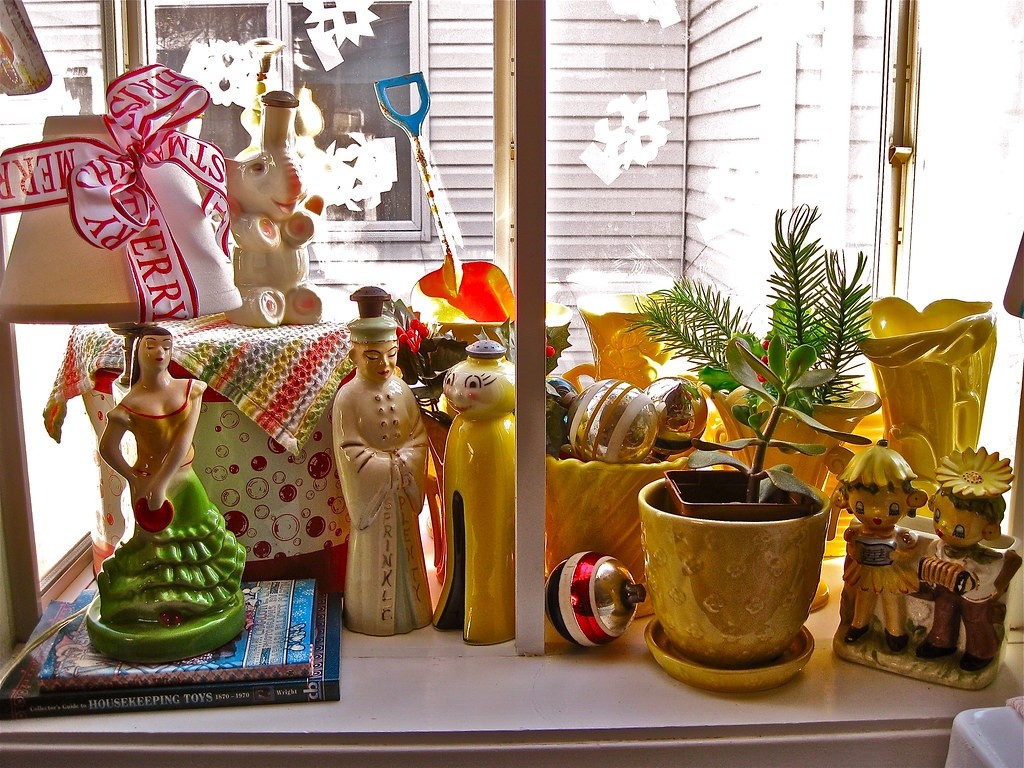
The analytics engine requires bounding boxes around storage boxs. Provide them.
[43,313,358,598]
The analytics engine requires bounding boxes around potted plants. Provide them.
[639,204,883,692]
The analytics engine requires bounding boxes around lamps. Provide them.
[0,63,246,548]
